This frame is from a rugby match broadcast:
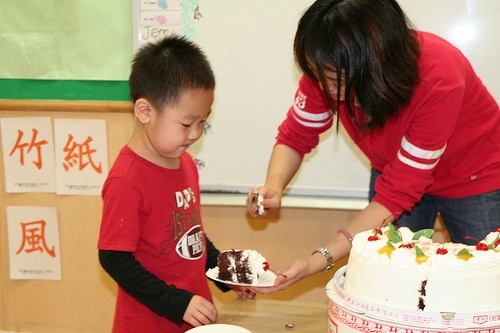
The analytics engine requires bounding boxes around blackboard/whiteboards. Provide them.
[132,1,499,198]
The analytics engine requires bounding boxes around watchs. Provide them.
[311,247,335,274]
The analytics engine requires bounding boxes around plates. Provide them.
[184,324,251,333]
[206,268,277,287]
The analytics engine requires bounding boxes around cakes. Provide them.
[340,215,500,315]
[218,248,270,284]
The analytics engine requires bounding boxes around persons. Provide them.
[95,35,257,333]
[244,0,500,296]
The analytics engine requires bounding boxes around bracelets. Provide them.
[337,228,354,245]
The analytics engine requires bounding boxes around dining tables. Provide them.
[209,295,328,333]
